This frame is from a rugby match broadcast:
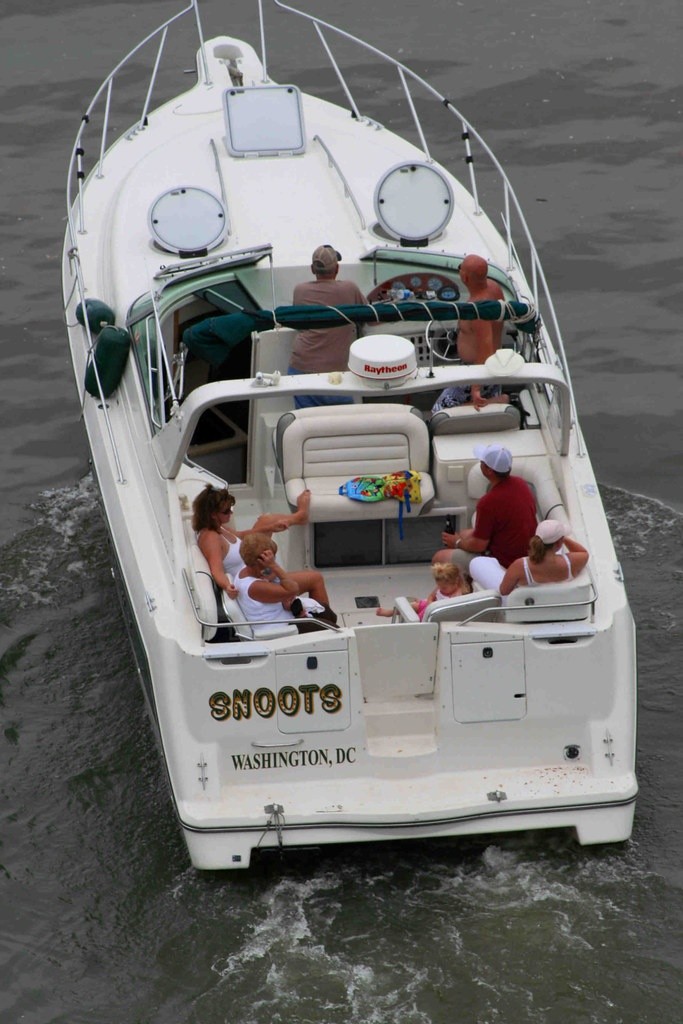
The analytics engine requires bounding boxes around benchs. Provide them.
[466,456,592,622]
[178,300,540,449]
[177,478,338,643]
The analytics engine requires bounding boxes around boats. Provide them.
[60,0,641,874]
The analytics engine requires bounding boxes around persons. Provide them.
[233,532,337,634]
[376,563,469,622]
[287,245,380,409]
[430,255,509,412]
[191,484,329,606]
[432,444,538,580]
[470,520,589,606]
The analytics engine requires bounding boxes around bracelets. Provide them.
[455,539,460,548]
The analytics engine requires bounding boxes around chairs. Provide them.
[391,582,503,623]
[275,403,436,522]
[423,403,519,433]
